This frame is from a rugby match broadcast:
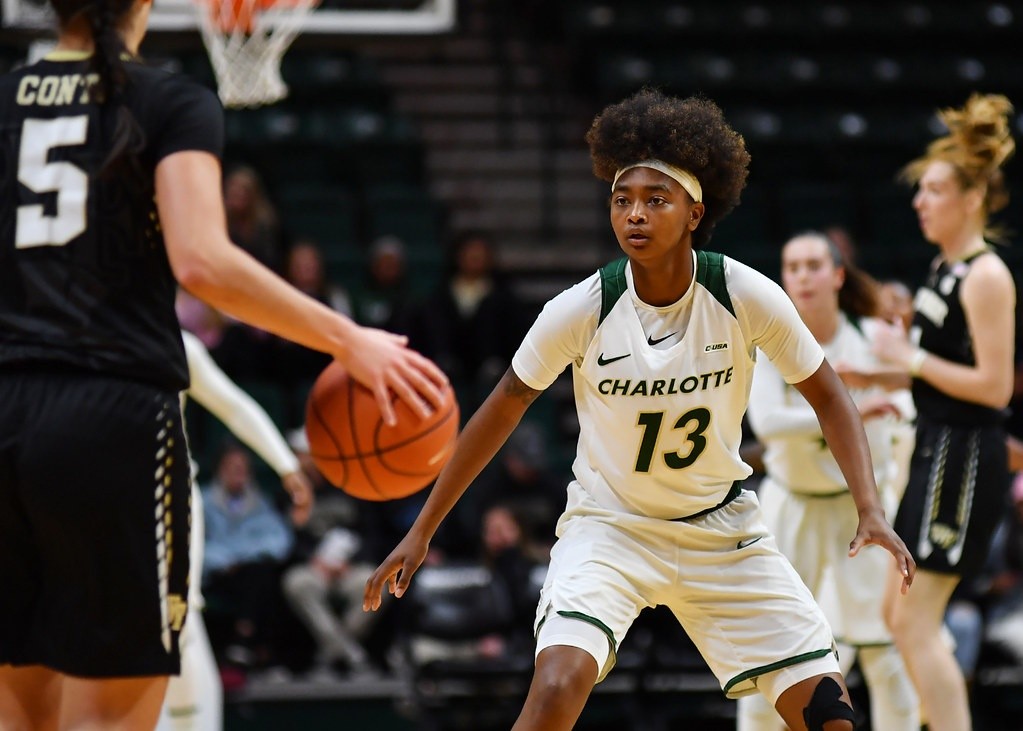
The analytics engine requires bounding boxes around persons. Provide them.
[157,320,312,731]
[175,157,680,713]
[858,93,1017,731]
[0,1,448,731]
[360,91,915,731]
[735,224,917,731]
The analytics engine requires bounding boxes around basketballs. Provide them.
[307,352,460,498]
